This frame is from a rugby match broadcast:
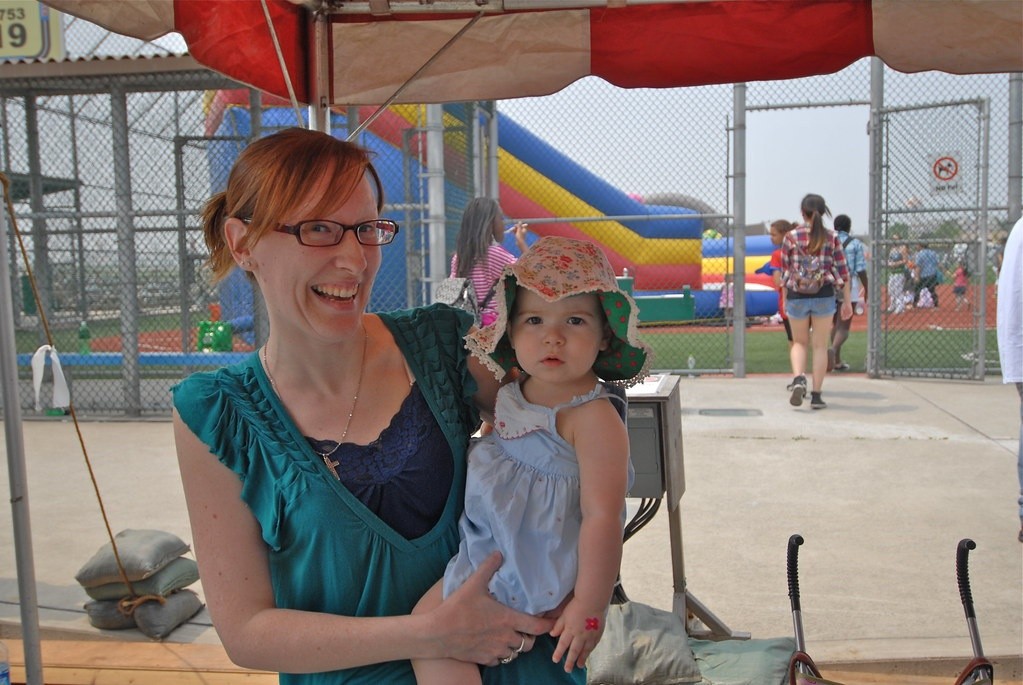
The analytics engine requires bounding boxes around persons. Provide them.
[718,194,968,409]
[404,238,658,685]
[450,196,534,307]
[169,125,586,685]
[996,212,1023,543]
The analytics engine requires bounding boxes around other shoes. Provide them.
[811,390,826,408]
[834,362,850,370]
[787,378,806,390]
[790,376,803,405]
[887,305,904,313]
[827,346,835,372]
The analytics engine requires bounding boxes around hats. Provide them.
[464,236,657,388]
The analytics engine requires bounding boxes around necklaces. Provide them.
[265,322,366,482]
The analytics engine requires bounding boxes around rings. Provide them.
[514,634,526,654]
[499,650,516,663]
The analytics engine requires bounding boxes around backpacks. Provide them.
[786,229,824,294]
[433,276,502,317]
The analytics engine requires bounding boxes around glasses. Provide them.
[243,215,399,247]
